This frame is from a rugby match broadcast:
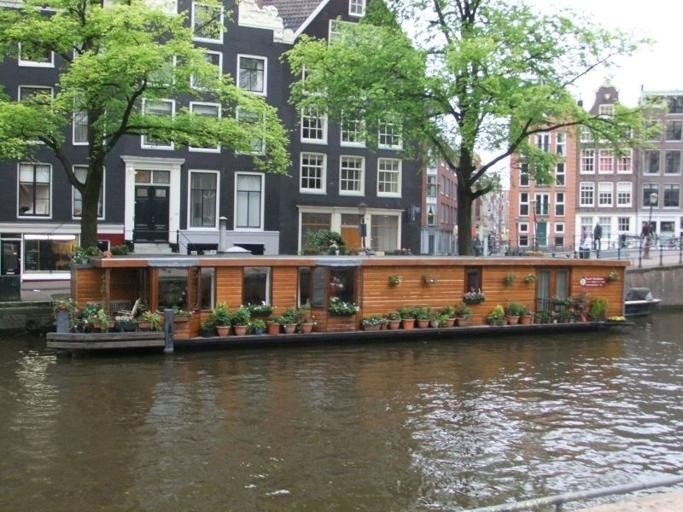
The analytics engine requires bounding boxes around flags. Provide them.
[532,207,538,224]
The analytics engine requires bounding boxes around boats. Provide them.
[624,285,665,317]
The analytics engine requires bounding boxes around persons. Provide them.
[647,222,654,236]
[453,222,496,256]
[594,222,602,251]
[641,221,647,237]
[580,226,587,248]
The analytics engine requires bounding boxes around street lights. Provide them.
[356,200,370,250]
[639,191,659,260]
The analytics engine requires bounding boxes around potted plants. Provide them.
[204,301,317,336]
[489,295,607,326]
[50,295,191,335]
[363,302,470,331]
